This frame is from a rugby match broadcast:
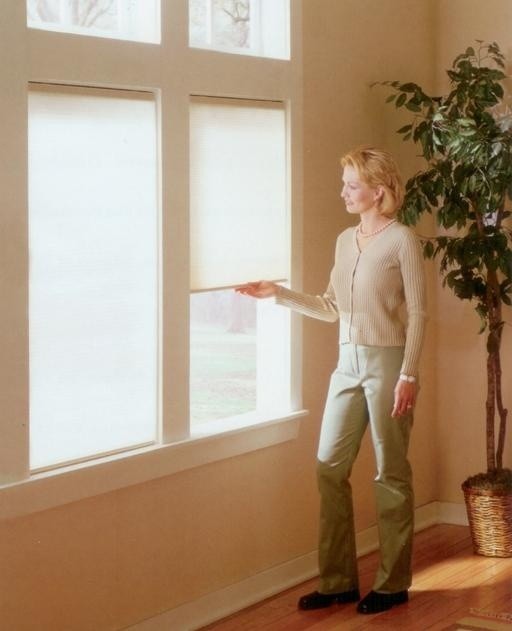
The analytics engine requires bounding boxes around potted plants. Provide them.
[368,34,512,556]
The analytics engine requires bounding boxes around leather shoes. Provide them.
[297,586,362,613]
[355,588,410,616]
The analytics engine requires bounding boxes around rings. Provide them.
[406,404,413,408]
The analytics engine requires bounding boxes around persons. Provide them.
[233,145,426,613]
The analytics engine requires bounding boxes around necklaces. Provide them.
[354,219,398,238]
